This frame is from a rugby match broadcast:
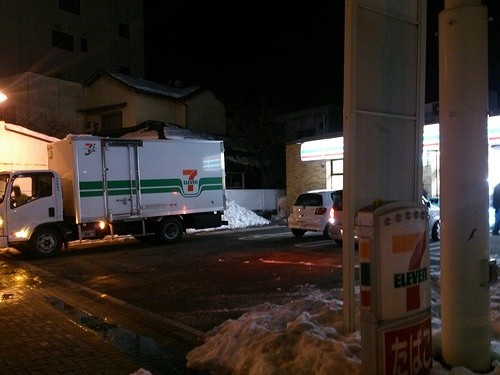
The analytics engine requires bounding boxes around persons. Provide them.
[13,185,35,207]
[492,182,500,236]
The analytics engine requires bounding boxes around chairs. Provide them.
[38,182,51,198]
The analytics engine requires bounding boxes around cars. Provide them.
[329,190,441,244]
[288,189,344,238]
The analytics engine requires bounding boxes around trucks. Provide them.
[0,134,228,259]
